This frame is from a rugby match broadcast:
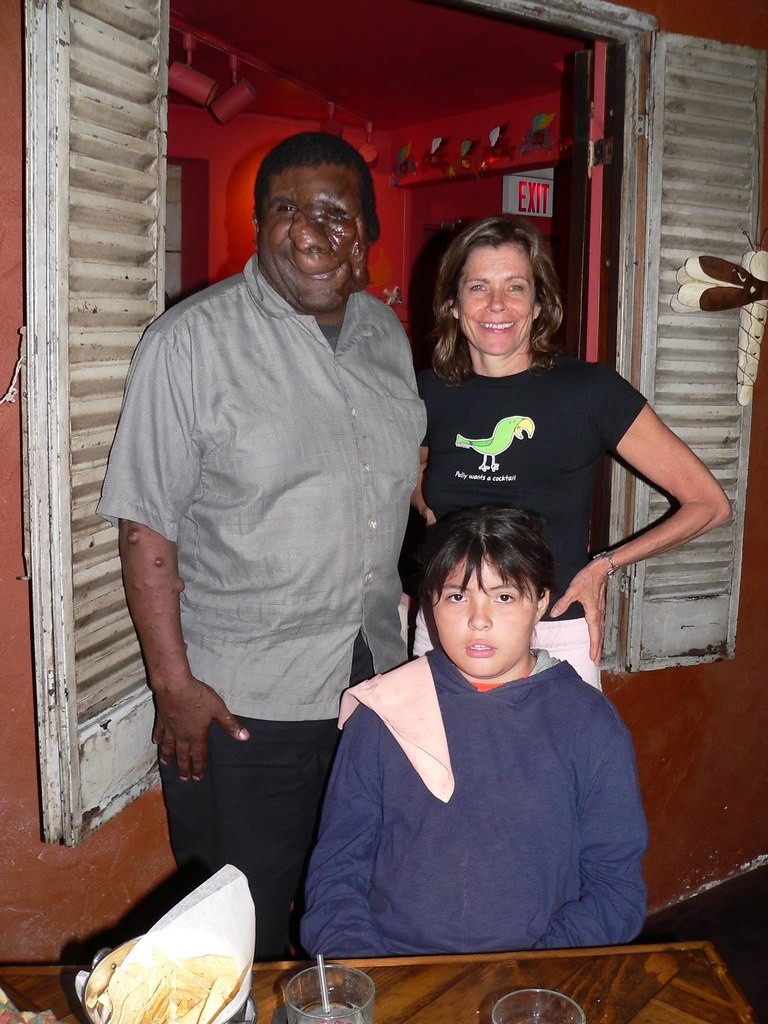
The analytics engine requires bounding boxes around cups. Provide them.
[492,989,586,1024]
[285,965,375,1024]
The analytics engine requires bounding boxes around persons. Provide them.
[94,133,432,961]
[302,501,650,960]
[398,215,733,691]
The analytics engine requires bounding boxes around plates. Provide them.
[85,935,252,1024]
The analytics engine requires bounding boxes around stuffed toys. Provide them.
[671,226,768,406]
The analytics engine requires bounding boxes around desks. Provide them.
[0,941,755,1024]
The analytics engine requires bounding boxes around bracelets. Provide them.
[592,549,620,577]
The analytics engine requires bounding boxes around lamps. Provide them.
[319,100,344,137]
[360,122,378,167]
[209,56,261,124]
[169,34,219,107]
